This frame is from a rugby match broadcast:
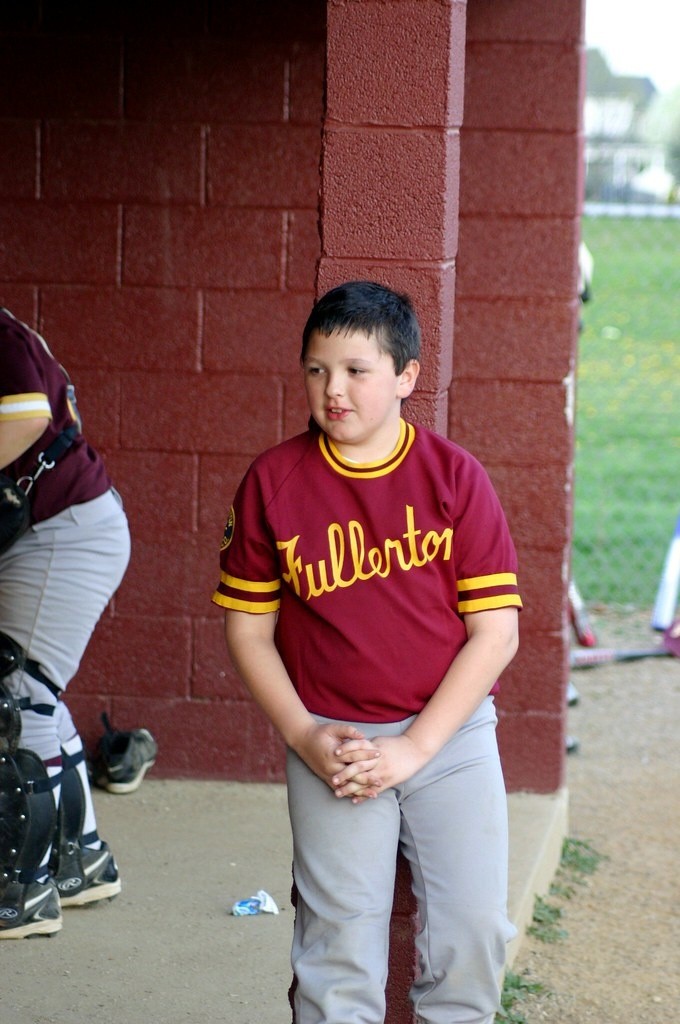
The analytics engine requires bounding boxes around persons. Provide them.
[212,278,523,1024]
[0,305,133,938]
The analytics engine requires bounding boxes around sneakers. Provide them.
[0,876,62,940]
[50,841,122,908]
[99,727,158,794]
